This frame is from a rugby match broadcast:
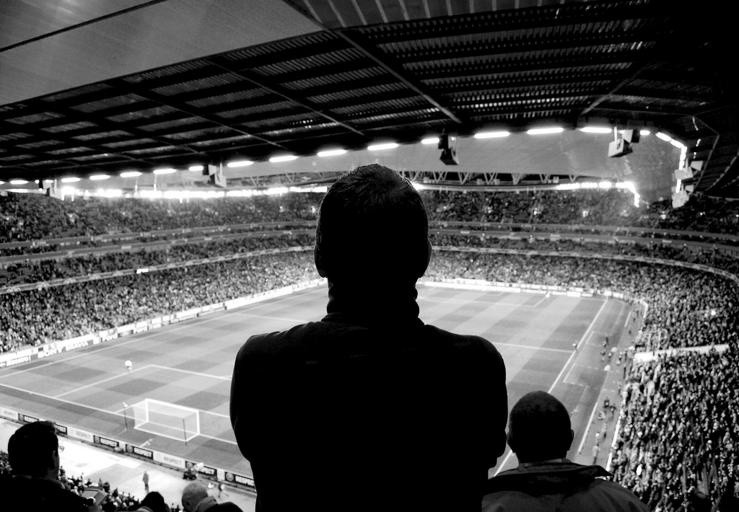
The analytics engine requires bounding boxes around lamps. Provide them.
[607,116,632,159]
[673,163,699,187]
[206,163,227,190]
[436,133,460,165]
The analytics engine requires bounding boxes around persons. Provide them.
[478,390,654,512]
[414,187,738,307]
[0,189,326,356]
[584,305,739,512]
[226,161,511,511]
[0,451,184,511]
[217,483,230,498]
[0,419,95,511]
[181,483,244,512]
